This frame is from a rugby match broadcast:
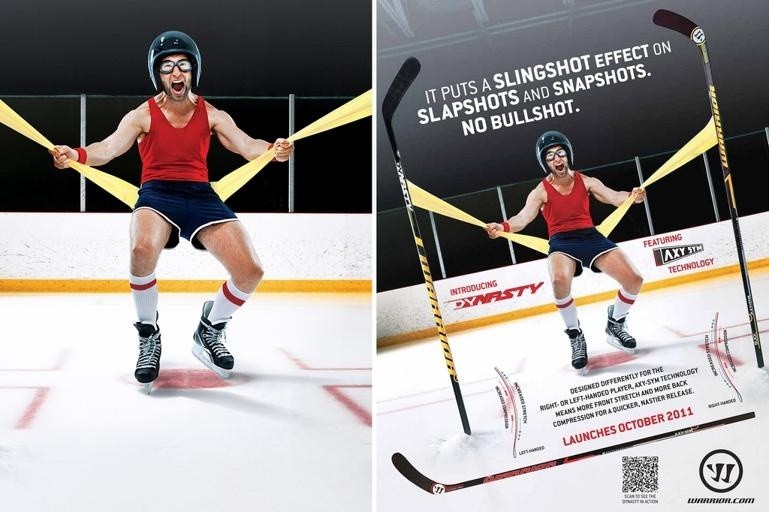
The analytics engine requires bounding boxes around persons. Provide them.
[50,31,296,383]
[485,130,645,369]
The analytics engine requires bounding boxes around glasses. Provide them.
[158,59,192,74]
[544,148,567,161]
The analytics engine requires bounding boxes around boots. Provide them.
[133,310,161,383]
[564,328,588,369]
[605,304,636,348]
[192,301,234,369]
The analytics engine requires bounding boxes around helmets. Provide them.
[148,30,201,91]
[535,131,573,174]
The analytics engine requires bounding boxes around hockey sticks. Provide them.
[382,56,471,435]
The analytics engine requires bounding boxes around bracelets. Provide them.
[500,220,512,235]
[266,142,276,163]
[628,193,636,205]
[74,146,87,164]
